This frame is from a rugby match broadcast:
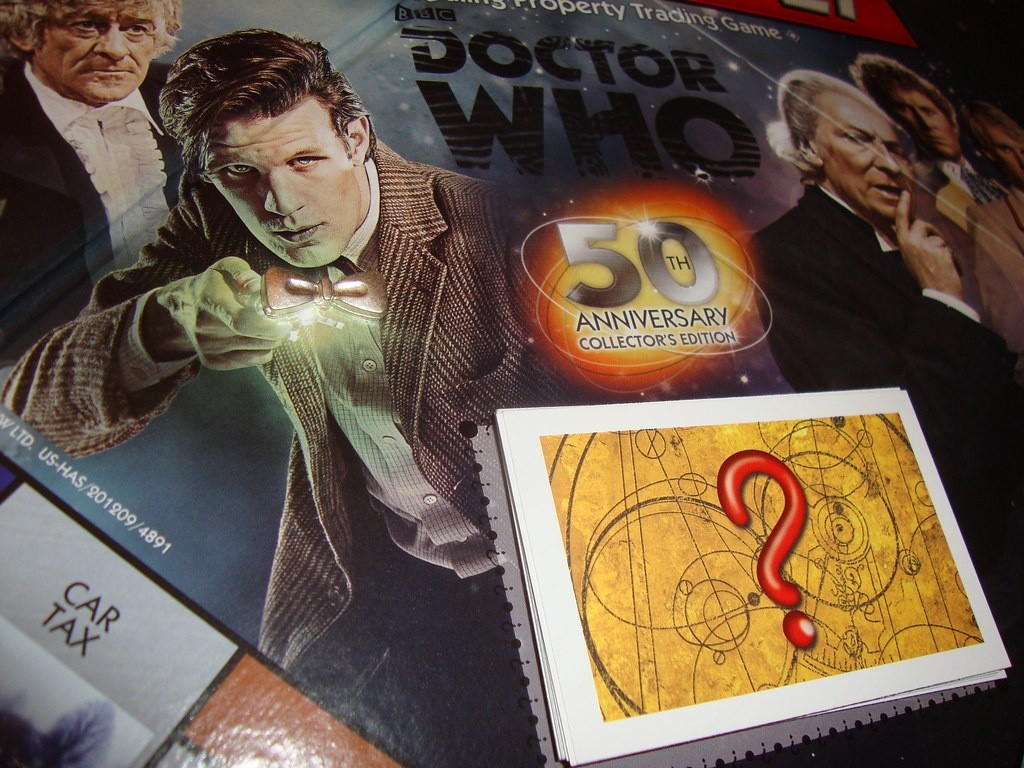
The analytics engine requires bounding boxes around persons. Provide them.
[751,50,1024,631]
[0,0,569,767]
